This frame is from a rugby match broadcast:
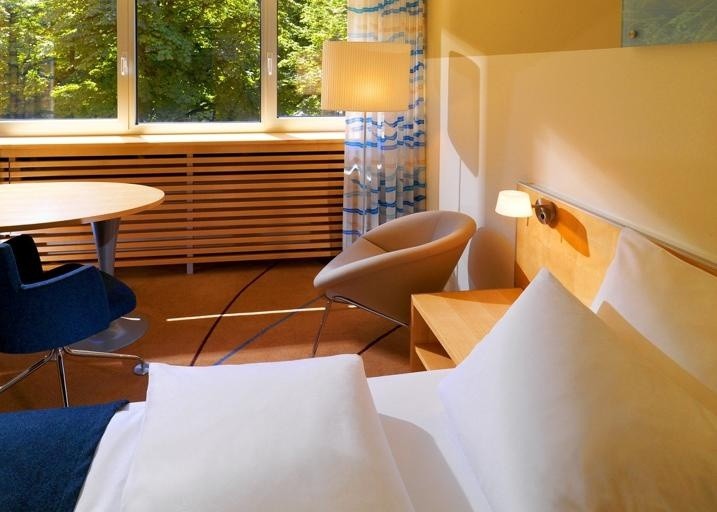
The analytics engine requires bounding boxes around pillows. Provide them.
[450,270,712,510]
[148,352,408,512]
[592,230,715,383]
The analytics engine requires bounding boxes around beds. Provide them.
[3,369,715,512]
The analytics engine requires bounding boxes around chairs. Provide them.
[0,234,149,407]
[311,210,477,356]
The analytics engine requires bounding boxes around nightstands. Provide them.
[409,287,526,372]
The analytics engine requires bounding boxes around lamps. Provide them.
[321,40,410,232]
[494,189,556,225]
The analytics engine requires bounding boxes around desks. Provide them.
[0,181,166,352]
